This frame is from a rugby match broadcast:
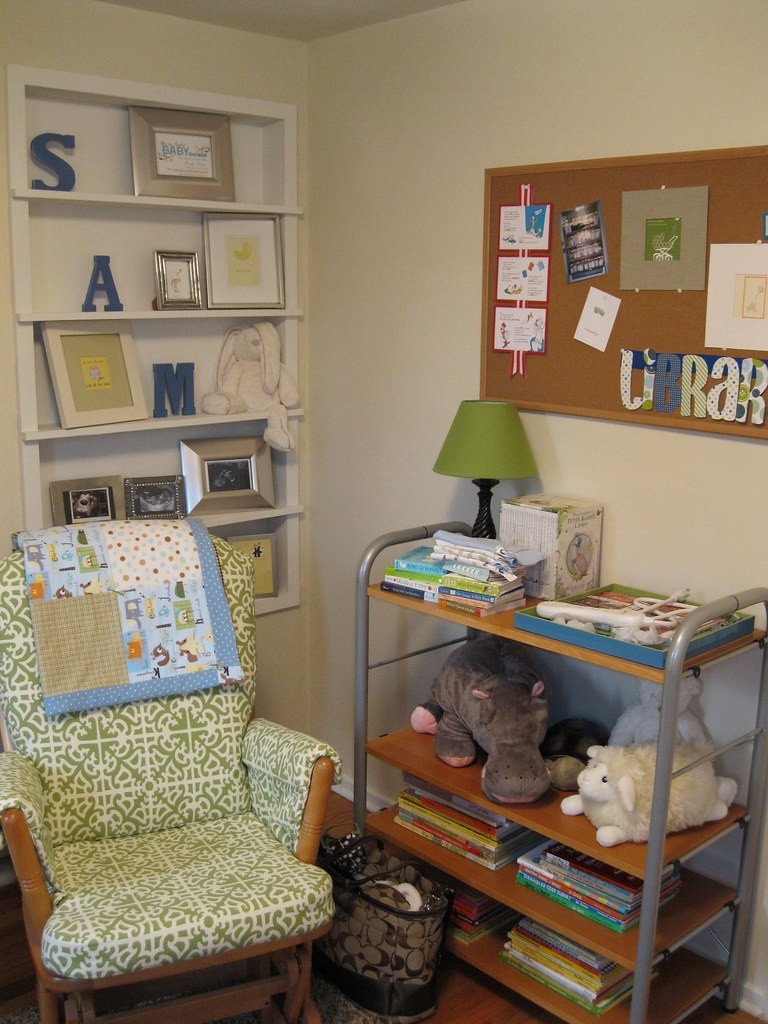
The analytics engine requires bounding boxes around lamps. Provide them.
[433,399,539,544]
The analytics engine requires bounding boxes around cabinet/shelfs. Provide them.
[346,519,768,1023]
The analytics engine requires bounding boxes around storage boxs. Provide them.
[498,493,602,604]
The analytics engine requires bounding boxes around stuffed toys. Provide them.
[200,320,299,452]
[407,634,738,846]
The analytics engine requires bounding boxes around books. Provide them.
[393,772,683,1016]
[380,544,527,618]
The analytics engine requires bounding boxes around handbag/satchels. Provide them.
[317,820,453,1023]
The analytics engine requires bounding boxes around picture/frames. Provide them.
[177,436,278,515]
[154,248,204,310]
[226,535,278,602]
[48,475,125,529]
[38,320,149,431]
[202,210,284,309]
[124,474,186,524]
[126,103,235,205]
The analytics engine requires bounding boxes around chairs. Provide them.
[1,516,344,1024]
